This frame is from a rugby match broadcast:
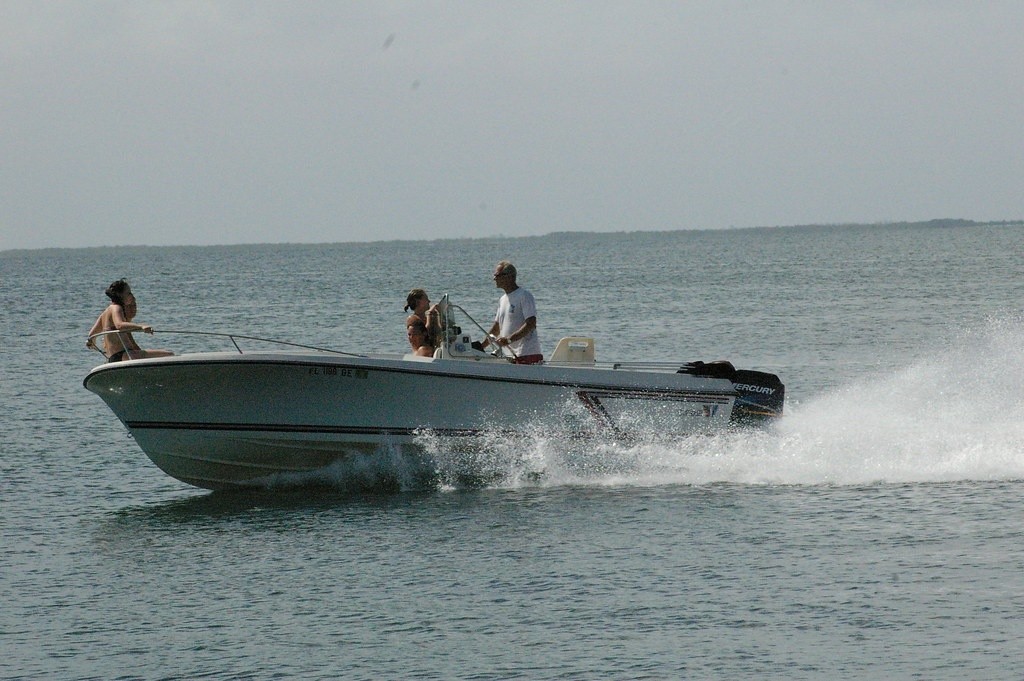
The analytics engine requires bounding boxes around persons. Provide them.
[404,288,441,357]
[482,260,543,365]
[86,277,175,363]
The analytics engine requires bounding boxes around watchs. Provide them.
[506,337,512,344]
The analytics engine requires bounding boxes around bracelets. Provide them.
[87,338,92,342]
[427,311,433,315]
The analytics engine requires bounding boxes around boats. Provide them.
[82,292,787,494]
